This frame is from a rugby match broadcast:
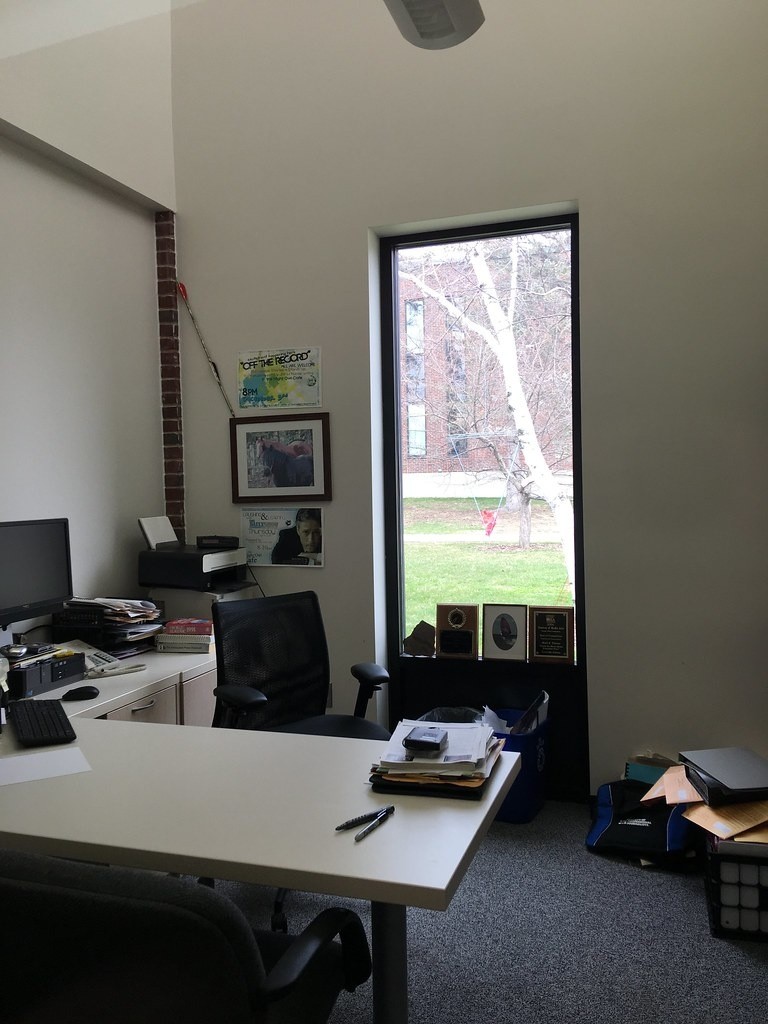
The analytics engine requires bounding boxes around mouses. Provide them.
[62,686,99,700]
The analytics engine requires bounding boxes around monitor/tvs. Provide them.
[0,518,74,626]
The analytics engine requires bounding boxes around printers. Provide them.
[138,515,258,595]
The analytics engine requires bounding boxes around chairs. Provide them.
[211,591,392,741]
[0,849,373,1024]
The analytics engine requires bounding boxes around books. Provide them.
[155,619,212,654]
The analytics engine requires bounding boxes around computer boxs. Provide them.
[8,652,86,700]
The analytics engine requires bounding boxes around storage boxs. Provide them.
[704,829,768,938]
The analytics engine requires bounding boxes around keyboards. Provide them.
[6,700,76,749]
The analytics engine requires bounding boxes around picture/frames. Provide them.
[482,603,527,663]
[229,412,332,504]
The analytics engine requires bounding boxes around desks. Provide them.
[0,717,522,1024]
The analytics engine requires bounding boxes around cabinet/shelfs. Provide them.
[0,618,217,727]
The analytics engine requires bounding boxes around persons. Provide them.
[270,509,323,566]
